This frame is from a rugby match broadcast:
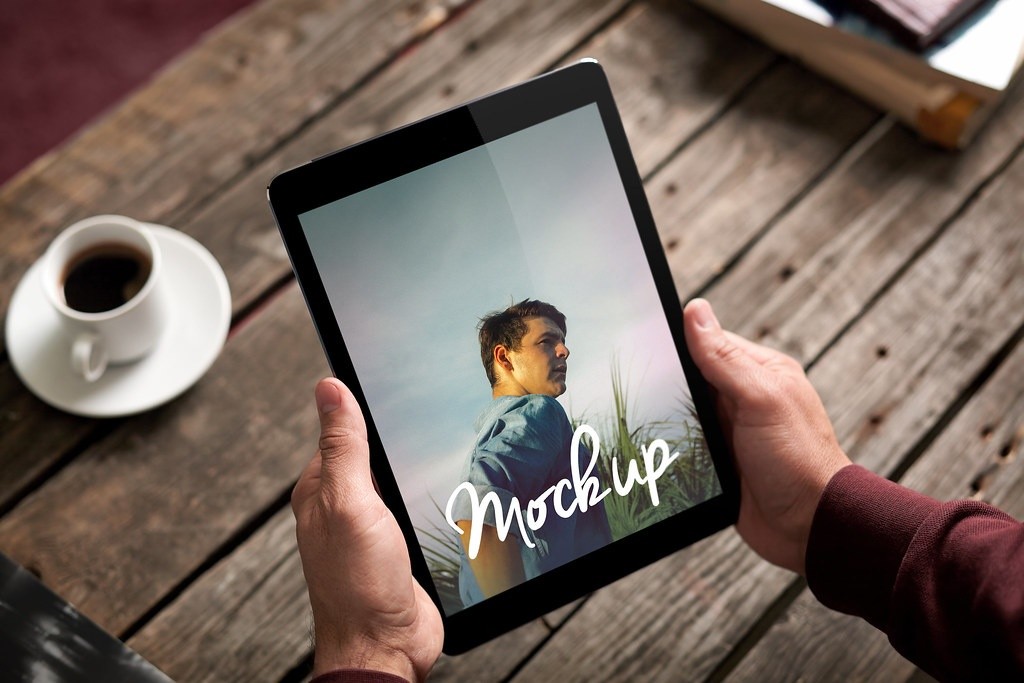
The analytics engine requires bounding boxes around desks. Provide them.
[0,0,1024,683]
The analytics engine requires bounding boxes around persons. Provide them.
[451,298,613,607]
[292,298,1024,682]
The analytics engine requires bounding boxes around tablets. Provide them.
[268,58,743,656]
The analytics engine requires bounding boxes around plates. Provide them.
[6,223,232,417]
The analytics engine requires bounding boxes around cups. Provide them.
[44,215,169,382]
